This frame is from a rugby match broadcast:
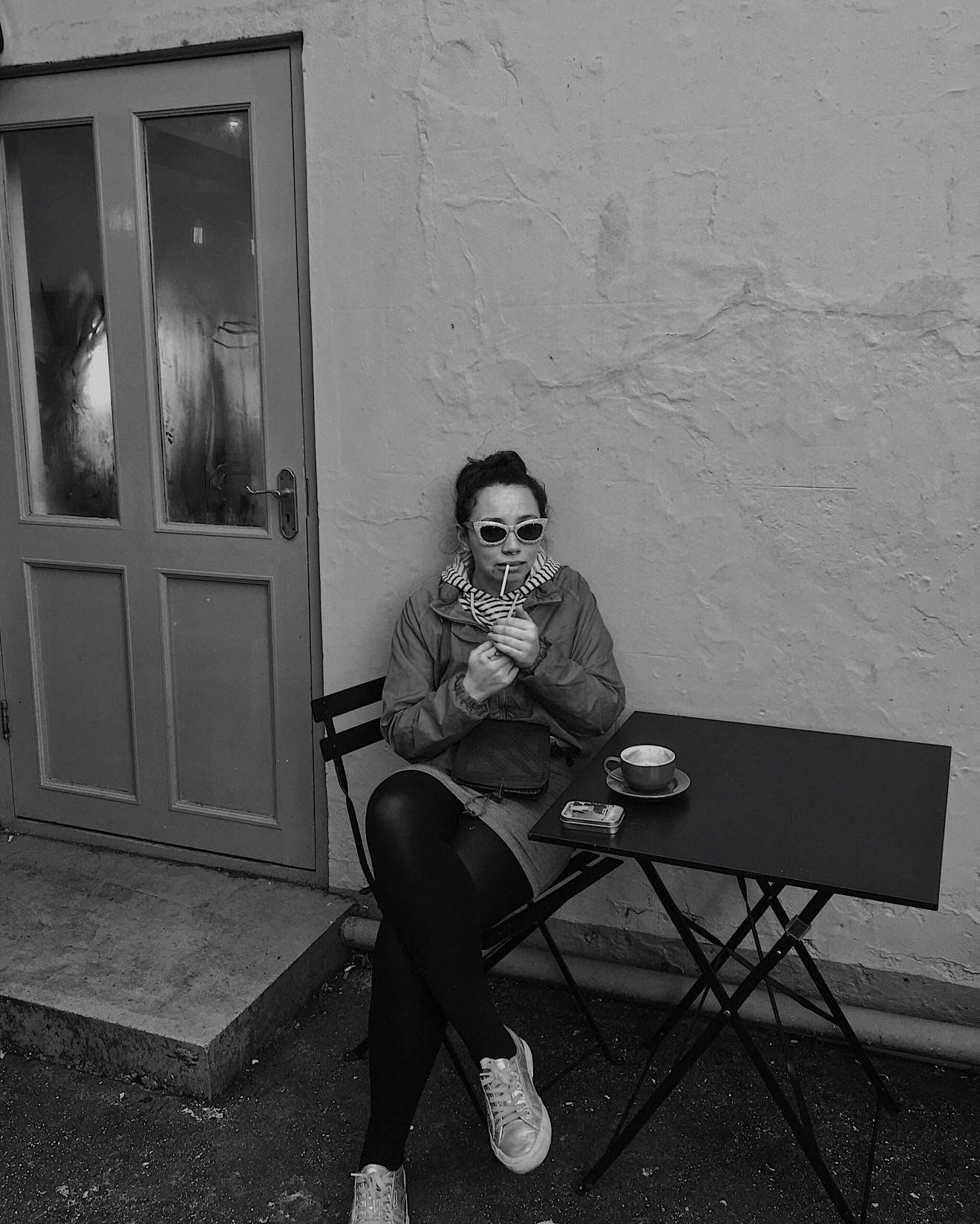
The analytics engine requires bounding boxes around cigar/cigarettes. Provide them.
[500,565,509,597]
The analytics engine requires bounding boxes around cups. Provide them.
[601,744,676,792]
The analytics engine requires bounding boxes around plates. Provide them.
[606,763,690,798]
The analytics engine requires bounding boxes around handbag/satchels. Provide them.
[452,718,552,795]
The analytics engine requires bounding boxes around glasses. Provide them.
[462,518,548,546]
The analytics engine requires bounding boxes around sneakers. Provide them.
[347,1164,410,1224]
[478,1025,552,1174]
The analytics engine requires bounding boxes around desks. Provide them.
[527,711,952,1224]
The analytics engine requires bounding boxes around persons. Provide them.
[348,451,626,1224]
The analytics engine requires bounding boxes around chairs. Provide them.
[309,673,620,1119]
